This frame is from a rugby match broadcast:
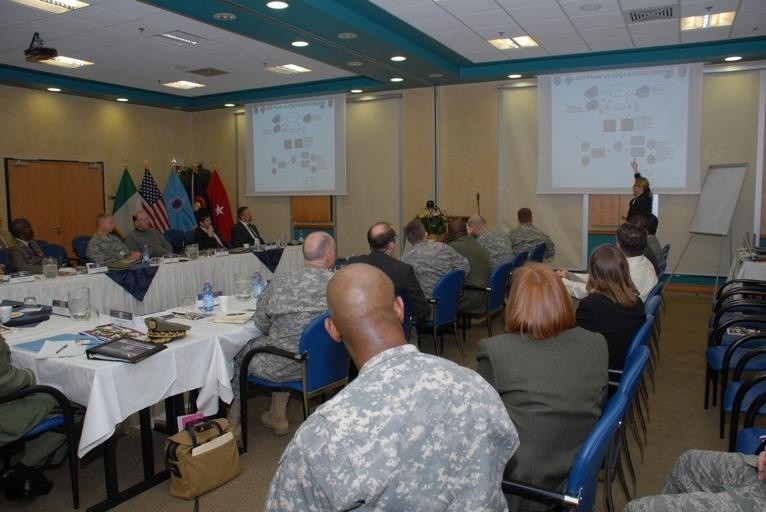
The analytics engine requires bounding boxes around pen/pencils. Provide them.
[227,313,245,315]
[56,344,68,353]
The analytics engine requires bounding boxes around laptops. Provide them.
[743,230,766,261]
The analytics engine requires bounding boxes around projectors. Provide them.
[24,38,57,63]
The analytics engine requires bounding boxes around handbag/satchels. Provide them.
[164,418,243,501]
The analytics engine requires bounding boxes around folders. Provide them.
[86,336,168,364]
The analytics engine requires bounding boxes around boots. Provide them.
[260,398,290,436]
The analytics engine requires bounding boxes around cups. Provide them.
[185,243,201,260]
[243,243,250,249]
[218,295,231,312]
[1,305,13,322]
[65,286,93,321]
[232,272,251,301]
[40,258,58,279]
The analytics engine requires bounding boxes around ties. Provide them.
[247,224,257,237]
[29,242,41,258]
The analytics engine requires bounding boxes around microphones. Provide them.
[477,193,481,216]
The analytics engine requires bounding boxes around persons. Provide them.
[264,263,519,510]
[404,208,556,353]
[226,231,338,441]
[551,213,666,378]
[621,158,652,224]
[346,221,432,345]
[474,262,610,511]
[622,441,766,511]
[0,335,86,500]
[9,207,266,274]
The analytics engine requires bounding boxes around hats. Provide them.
[144,317,191,344]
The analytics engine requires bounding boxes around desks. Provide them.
[1,236,412,511]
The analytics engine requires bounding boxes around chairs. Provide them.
[412,269,463,358]
[701,274,766,456]
[498,243,670,512]
[457,261,512,342]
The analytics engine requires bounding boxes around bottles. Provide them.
[251,270,264,299]
[201,279,214,312]
[142,244,150,263]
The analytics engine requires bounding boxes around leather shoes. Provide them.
[17,478,52,502]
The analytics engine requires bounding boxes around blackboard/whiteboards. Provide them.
[688,161,747,237]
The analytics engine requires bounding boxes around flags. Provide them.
[110,163,234,245]
